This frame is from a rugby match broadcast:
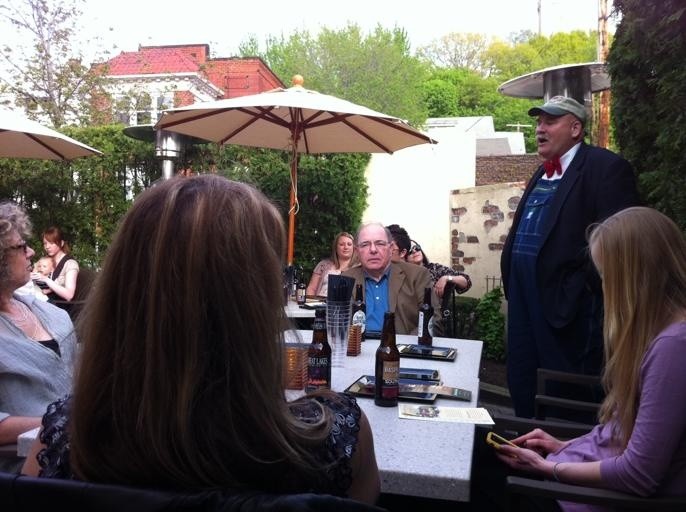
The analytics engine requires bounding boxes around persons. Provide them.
[469,206,684,510]
[19,174,381,510]
[341,221,447,337]
[405,241,472,335]
[306,232,362,298]
[500,95,640,418]
[34,256,65,304]
[1,203,79,473]
[30,223,79,321]
[385,223,411,262]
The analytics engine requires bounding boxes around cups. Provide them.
[281,343,311,391]
[348,323,363,356]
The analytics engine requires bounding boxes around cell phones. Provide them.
[486,431,519,458]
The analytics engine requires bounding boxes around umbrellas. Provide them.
[152,74,440,265]
[0,111,105,165]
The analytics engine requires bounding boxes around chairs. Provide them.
[0,471,391,512]
[530,367,604,424]
[436,283,457,338]
[492,413,686,511]
[50,267,98,324]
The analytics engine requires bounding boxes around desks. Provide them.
[283,291,329,318]
[281,327,485,504]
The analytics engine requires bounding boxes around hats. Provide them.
[528,95,588,130]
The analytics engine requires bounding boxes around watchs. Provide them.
[448,275,453,286]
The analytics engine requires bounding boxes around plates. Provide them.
[343,342,459,404]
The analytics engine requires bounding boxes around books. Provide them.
[343,375,446,404]
[396,343,459,362]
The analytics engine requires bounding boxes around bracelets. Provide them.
[552,462,563,482]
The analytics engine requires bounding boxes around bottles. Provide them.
[307,304,333,393]
[417,286,436,346]
[298,280,307,304]
[353,283,368,342]
[281,279,290,308]
[375,311,401,408]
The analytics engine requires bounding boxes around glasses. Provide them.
[407,244,421,255]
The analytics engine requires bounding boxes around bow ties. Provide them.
[542,153,563,178]
